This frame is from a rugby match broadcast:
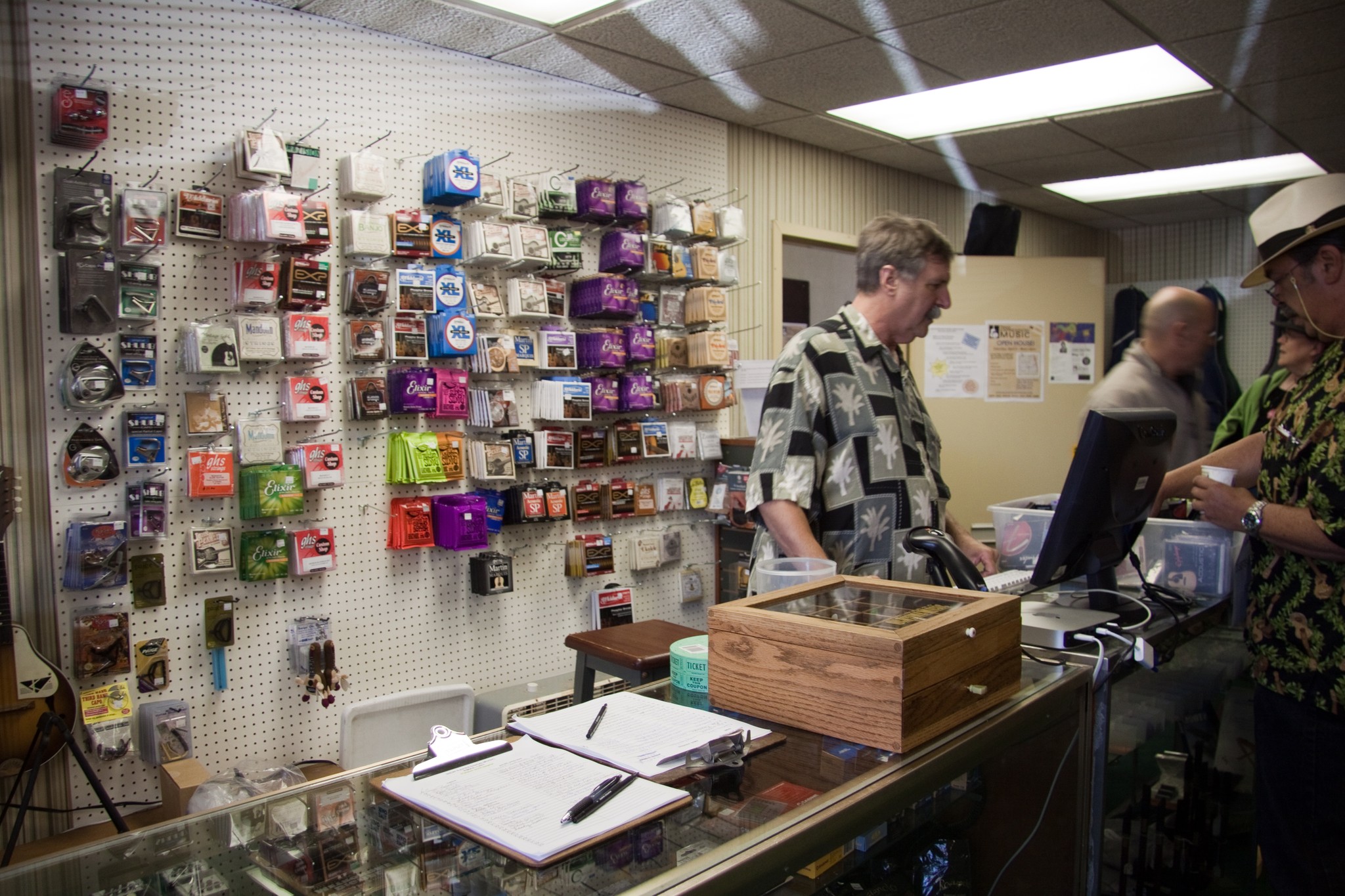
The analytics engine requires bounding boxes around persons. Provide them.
[1082,170,1345,896]
[744,214,1000,600]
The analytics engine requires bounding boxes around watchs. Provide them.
[1241,500,1269,536]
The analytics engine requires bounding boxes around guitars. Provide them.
[0,465,77,778]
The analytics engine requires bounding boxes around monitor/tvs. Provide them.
[1032,404,1179,618]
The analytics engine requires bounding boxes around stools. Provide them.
[565,620,708,707]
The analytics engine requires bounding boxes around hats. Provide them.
[1269,319,1316,340]
[1239,172,1345,291]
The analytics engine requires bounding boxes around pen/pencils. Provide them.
[586,703,607,739]
[572,772,639,823]
[561,774,622,824]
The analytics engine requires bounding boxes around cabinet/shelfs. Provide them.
[714,513,756,604]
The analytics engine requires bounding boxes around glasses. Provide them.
[1265,254,1315,306]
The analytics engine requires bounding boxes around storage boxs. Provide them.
[708,575,1022,753]
[986,493,1245,598]
[159,757,211,817]
